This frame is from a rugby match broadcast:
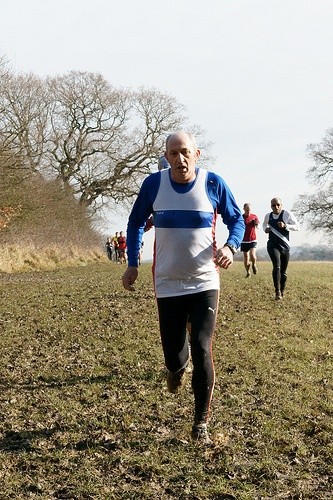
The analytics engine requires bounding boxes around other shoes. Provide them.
[252,265,257,275]
[167,369,185,395]
[275,290,283,299]
[191,424,214,445]
[245,274,250,278]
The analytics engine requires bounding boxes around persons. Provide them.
[117,231,127,264]
[262,198,300,300]
[110,232,120,261]
[105,237,114,261]
[139,241,144,265]
[122,131,246,445]
[241,203,260,278]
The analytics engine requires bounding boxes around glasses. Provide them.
[272,204,280,207]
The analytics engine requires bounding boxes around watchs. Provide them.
[224,243,237,255]
[284,224,287,229]
